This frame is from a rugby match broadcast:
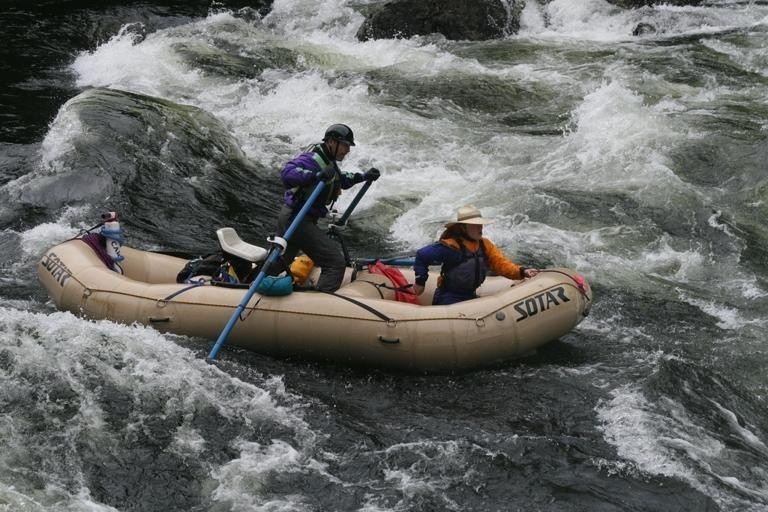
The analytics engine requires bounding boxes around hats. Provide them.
[444,204,494,228]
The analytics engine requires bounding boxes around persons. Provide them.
[413,204,540,306]
[242,122,382,294]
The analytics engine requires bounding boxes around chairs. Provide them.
[216,227,268,263]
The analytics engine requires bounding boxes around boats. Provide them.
[37,237,597,370]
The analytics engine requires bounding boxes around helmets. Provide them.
[321,123,355,146]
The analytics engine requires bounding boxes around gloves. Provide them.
[361,168,380,181]
[315,167,335,181]
